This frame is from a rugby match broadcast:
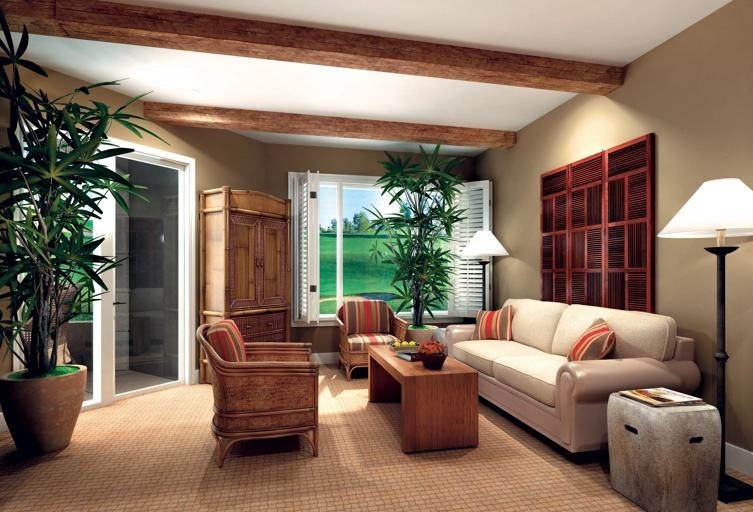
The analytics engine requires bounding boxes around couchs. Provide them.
[444,298,702,465]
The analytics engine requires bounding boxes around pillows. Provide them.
[206,318,247,363]
[343,299,390,335]
[567,317,615,362]
[470,304,513,341]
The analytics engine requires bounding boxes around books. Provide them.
[395,350,421,361]
[618,386,705,406]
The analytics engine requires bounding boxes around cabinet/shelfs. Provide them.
[199,185,292,384]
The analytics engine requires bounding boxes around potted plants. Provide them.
[368,141,469,344]
[0,12,171,463]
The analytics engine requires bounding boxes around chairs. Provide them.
[195,323,320,468]
[335,304,410,381]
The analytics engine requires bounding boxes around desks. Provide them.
[606,391,722,512]
